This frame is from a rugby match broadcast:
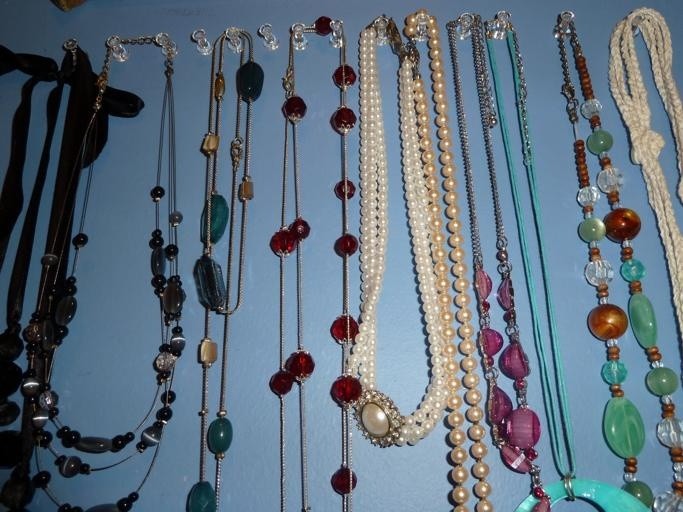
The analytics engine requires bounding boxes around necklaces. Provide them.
[608,10,683,334]
[0,44,61,512]
[447,14,553,512]
[556,18,683,512]
[486,12,648,512]
[188,26,265,512]
[22,32,188,512]
[403,10,493,512]
[348,16,447,449]
[270,15,363,512]
